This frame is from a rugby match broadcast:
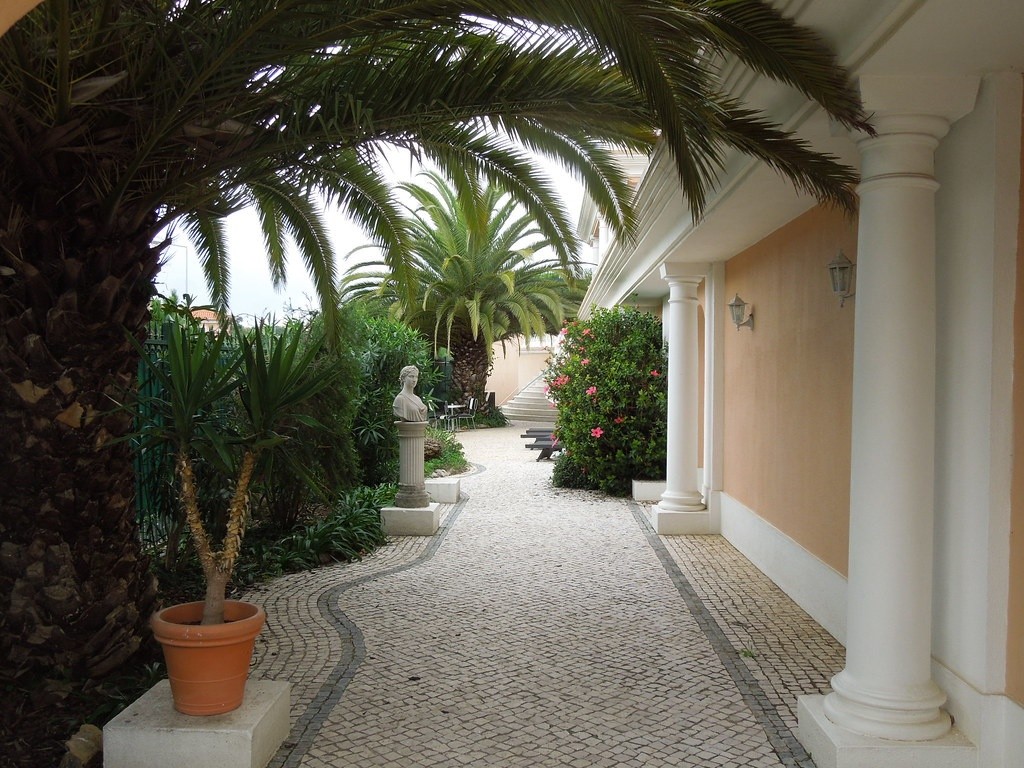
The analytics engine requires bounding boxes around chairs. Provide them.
[430,398,480,433]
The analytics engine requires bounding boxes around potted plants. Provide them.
[79,304,345,713]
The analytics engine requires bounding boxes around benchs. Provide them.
[520,428,563,462]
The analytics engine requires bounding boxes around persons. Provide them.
[392,365,428,421]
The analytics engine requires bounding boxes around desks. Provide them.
[443,405,465,432]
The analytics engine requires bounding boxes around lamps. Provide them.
[728,293,755,331]
[823,247,854,308]
